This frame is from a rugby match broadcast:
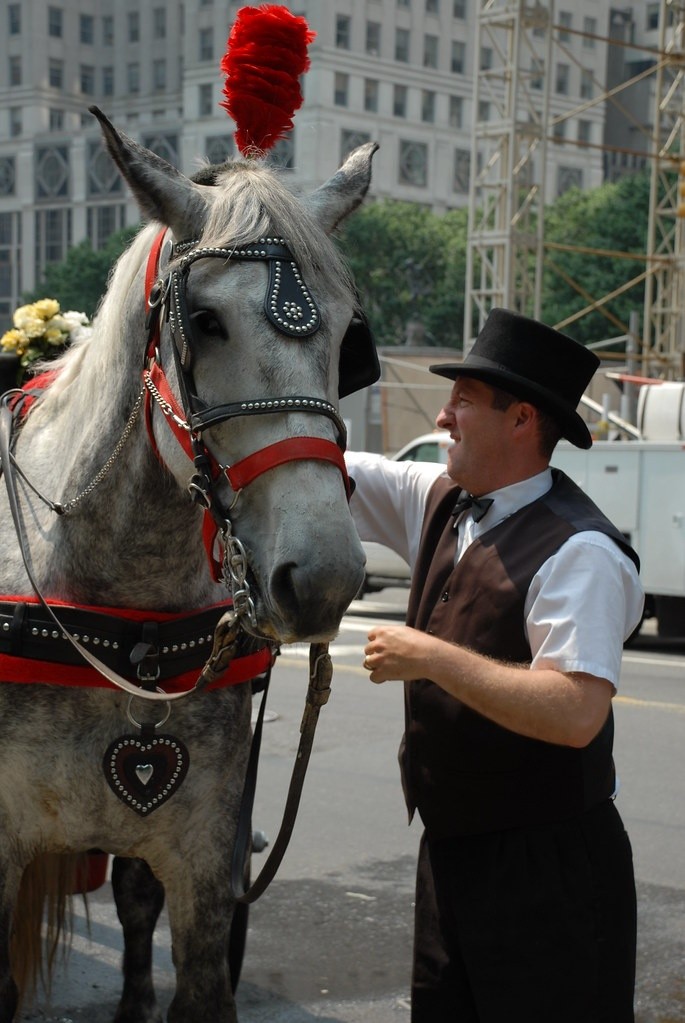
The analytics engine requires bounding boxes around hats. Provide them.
[428,308,601,449]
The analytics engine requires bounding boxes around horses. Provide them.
[1,105,380,1022]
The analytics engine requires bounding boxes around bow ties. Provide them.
[452,498,494,523]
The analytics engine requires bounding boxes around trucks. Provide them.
[361,431,685,648]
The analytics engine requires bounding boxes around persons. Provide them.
[343,306,646,1023]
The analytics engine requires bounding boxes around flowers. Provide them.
[0,297,92,372]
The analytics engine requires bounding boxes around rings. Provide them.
[364,655,372,670]
